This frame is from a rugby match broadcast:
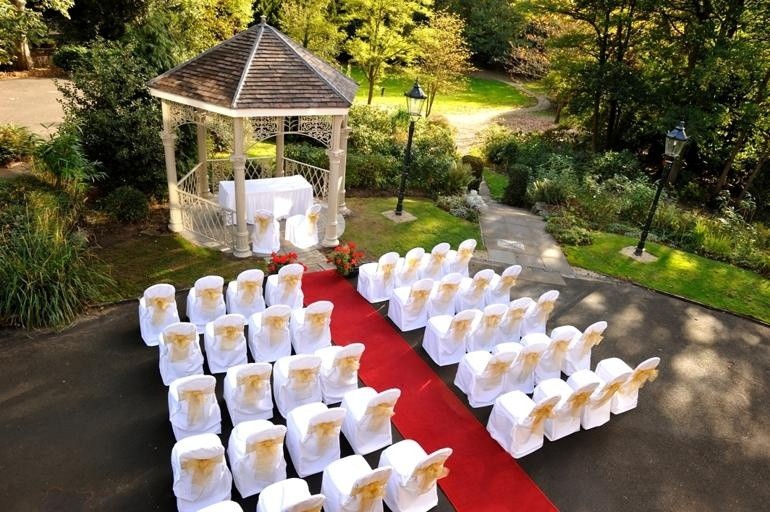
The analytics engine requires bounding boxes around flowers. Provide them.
[264,251,307,272]
[324,241,365,276]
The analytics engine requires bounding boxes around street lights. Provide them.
[395,75,429,216]
[635,119,691,260]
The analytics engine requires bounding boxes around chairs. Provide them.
[227,420,287,495]
[511,291,559,337]
[336,385,401,458]
[314,342,363,408]
[484,297,528,349]
[423,272,461,321]
[224,361,273,428]
[492,342,547,395]
[254,478,326,512]
[421,309,475,367]
[139,264,304,347]
[158,299,334,388]
[283,402,342,480]
[386,278,432,332]
[520,328,576,387]
[194,501,244,512]
[532,379,600,442]
[487,390,562,461]
[485,265,523,306]
[169,374,221,440]
[380,432,452,511]
[272,355,323,421]
[452,268,493,314]
[595,357,662,415]
[458,305,506,356]
[320,455,393,512]
[451,350,519,410]
[284,206,321,247]
[356,238,477,303]
[549,321,606,376]
[171,430,232,510]
[566,371,631,430]
[251,209,281,255]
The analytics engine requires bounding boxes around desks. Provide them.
[219,175,314,226]
